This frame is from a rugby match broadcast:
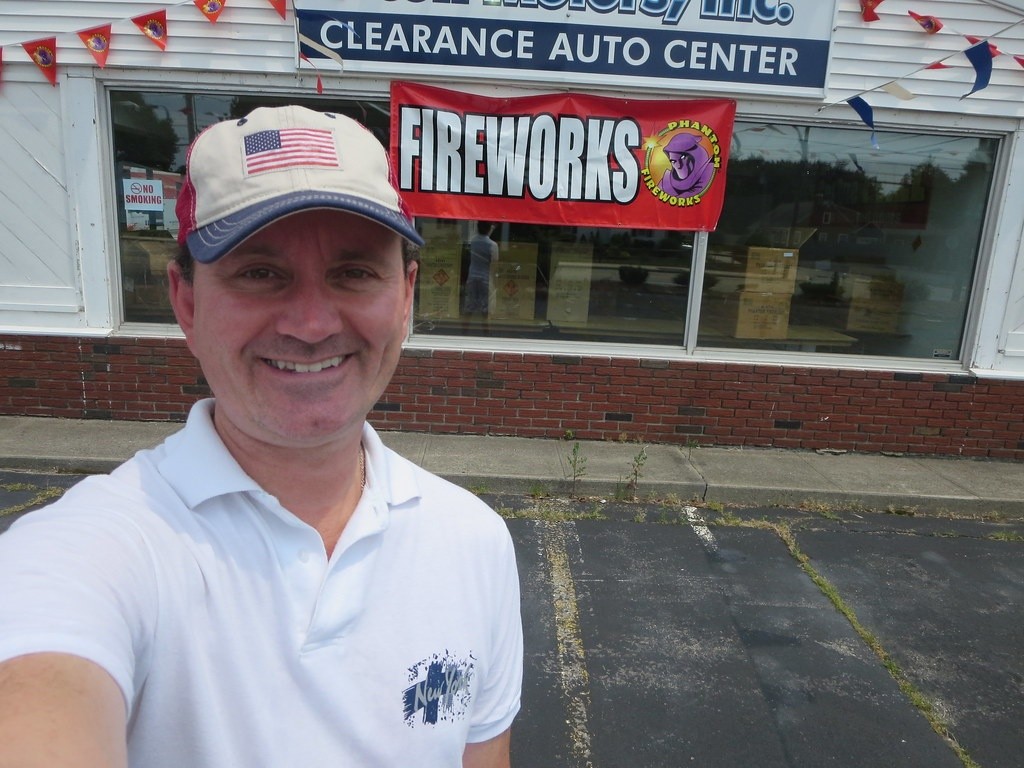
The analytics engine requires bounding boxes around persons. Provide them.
[0,105,524,768]
[463,220,500,335]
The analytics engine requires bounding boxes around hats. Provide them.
[176,105,425,264]
[478,221,495,228]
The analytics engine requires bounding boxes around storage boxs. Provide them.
[484,238,538,322]
[835,273,905,331]
[719,242,798,293]
[414,222,462,317]
[703,287,792,338]
[545,241,591,323]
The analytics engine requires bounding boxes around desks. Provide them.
[412,285,856,353]
